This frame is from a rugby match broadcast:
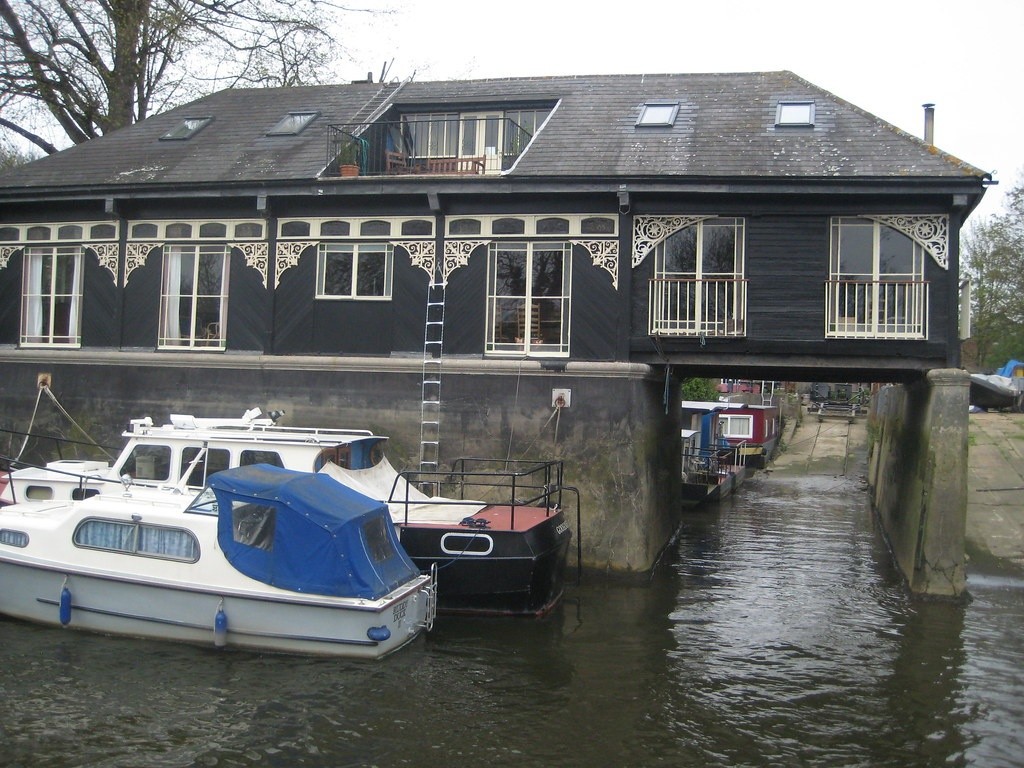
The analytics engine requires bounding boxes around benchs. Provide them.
[427,153,487,173]
[385,149,421,175]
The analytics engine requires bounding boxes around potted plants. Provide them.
[338,138,360,176]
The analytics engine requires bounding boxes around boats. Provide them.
[680,401,747,503]
[1,407,581,616]
[0,462,440,664]
